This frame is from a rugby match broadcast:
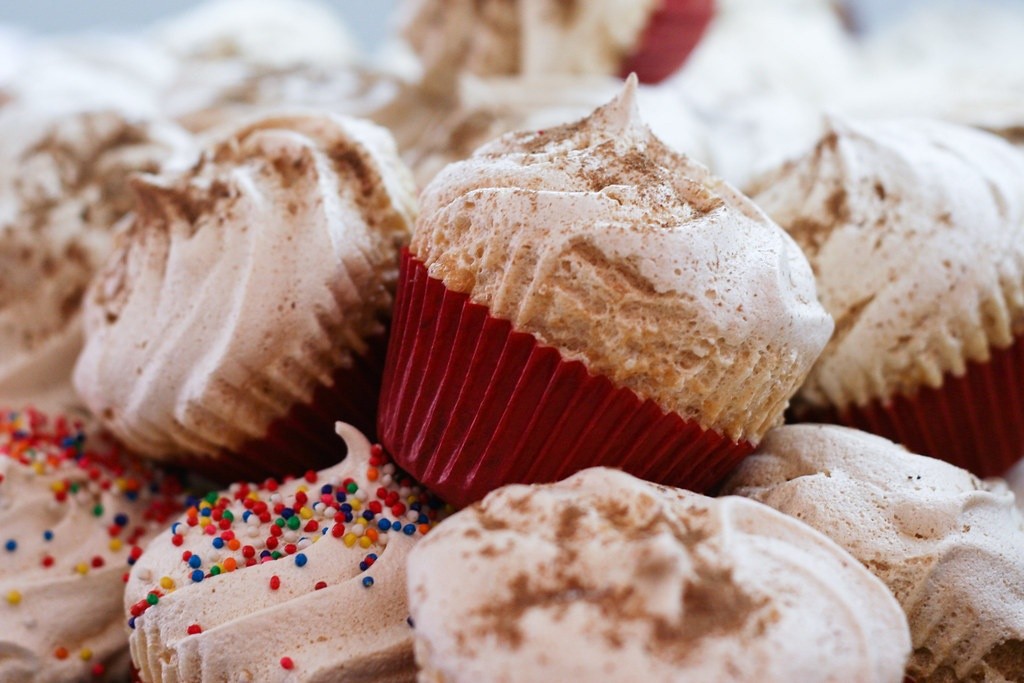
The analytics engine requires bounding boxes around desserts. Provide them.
[0,0,1024,683]
